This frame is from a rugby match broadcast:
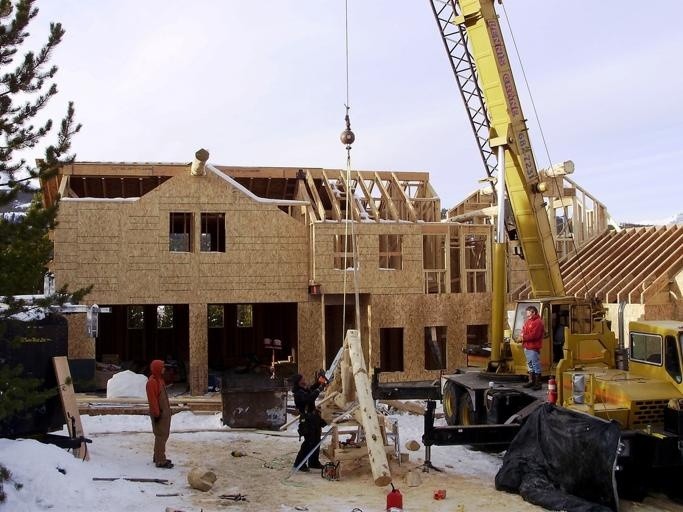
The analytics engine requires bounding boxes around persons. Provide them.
[288,372,334,472]
[146,359,175,468]
[516,306,544,391]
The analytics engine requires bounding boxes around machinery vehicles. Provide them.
[341,2,681,511]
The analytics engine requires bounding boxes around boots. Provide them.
[522,372,534,388]
[530,373,542,391]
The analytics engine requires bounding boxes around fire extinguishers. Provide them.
[547,375,557,407]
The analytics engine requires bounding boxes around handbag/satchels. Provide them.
[320,462,339,481]
[298,416,312,436]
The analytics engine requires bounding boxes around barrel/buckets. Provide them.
[264,338,272,345]
[274,339,281,345]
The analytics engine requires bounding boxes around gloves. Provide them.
[154,415,162,423]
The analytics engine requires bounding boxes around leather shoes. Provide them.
[294,462,311,472]
[153,457,172,462]
[307,461,324,469]
[156,461,175,468]
